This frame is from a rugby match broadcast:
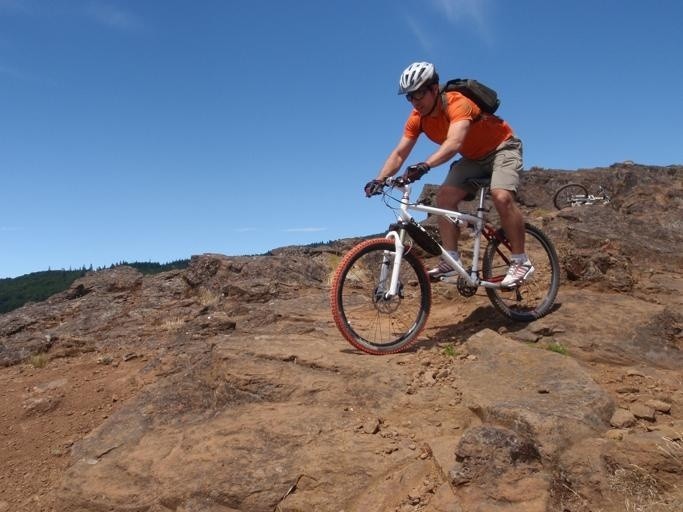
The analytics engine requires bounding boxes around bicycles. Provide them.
[330,168,561,358]
[552,183,612,211]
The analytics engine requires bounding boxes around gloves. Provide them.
[365,179,383,198]
[404,162,430,184]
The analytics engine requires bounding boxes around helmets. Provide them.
[399,62,434,95]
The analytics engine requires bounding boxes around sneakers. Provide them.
[427,258,462,277]
[501,259,535,288]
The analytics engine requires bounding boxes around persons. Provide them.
[365,60,535,288]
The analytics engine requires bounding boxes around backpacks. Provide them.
[440,79,500,112]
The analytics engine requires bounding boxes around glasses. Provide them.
[405,90,426,101]
